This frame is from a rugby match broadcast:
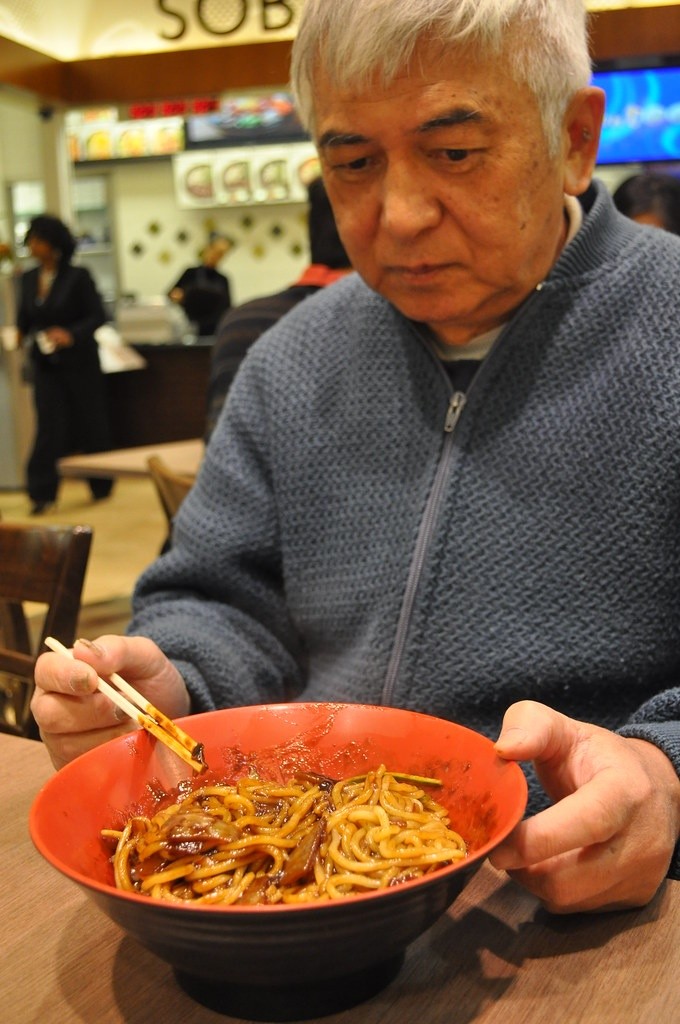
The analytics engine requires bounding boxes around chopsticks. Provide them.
[43,635,209,775]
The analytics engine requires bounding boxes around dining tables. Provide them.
[0,731,680,1024]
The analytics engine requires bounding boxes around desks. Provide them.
[58,429,207,480]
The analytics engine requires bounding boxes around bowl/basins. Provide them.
[28,703,528,988]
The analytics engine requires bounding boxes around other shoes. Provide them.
[32,499,52,515]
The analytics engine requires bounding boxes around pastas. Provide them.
[102,764,468,907]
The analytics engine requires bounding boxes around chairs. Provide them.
[0,522,94,741]
[148,456,196,523]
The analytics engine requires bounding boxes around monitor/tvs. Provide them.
[584,53,679,165]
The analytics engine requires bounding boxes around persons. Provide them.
[169,237,234,334]
[203,178,358,447]
[612,173,679,239]
[15,213,113,516]
[29,0,680,914]
